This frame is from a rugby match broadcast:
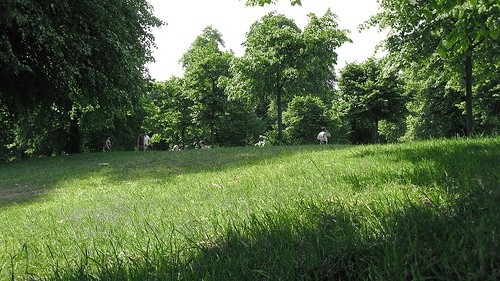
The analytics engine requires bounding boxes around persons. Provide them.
[138,131,145,151]
[142,131,151,151]
[316,126,331,144]
[105,135,111,152]
